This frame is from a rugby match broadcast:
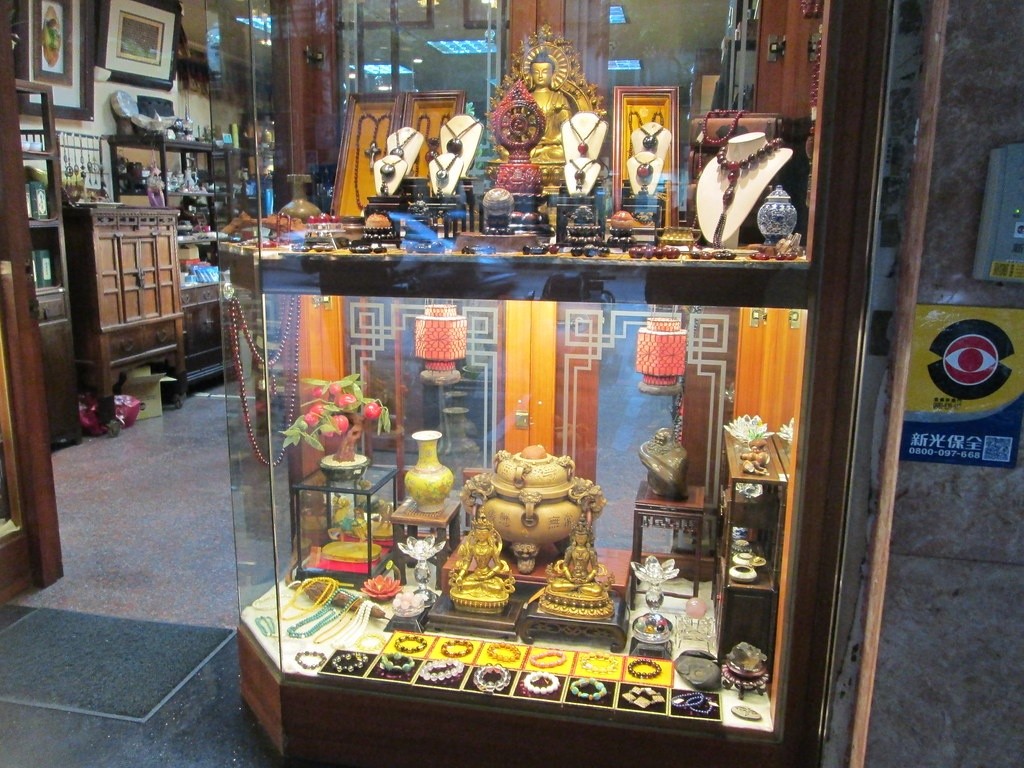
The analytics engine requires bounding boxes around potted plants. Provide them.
[278,373,391,480]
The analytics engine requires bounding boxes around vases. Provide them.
[404,431,454,512]
[207,22,221,80]
[436,406,479,490]
[277,173,322,224]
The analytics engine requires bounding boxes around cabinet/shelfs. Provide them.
[14,79,83,446]
[108,135,274,385]
[62,208,188,421]
[711,430,790,684]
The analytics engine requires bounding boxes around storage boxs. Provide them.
[119,365,178,420]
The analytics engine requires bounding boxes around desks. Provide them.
[630,480,707,610]
[389,498,461,590]
[441,534,632,607]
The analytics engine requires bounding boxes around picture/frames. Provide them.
[18,0,94,123]
[97,0,183,91]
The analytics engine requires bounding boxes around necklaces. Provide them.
[252,575,378,650]
[228,294,301,467]
[805,33,822,207]
[353,108,785,246]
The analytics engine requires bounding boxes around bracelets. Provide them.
[295,633,712,715]
[236,214,797,261]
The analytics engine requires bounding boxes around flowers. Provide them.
[361,575,402,601]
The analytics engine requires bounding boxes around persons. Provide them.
[498,51,572,164]
[455,523,506,597]
[639,426,688,497]
[550,519,604,597]
